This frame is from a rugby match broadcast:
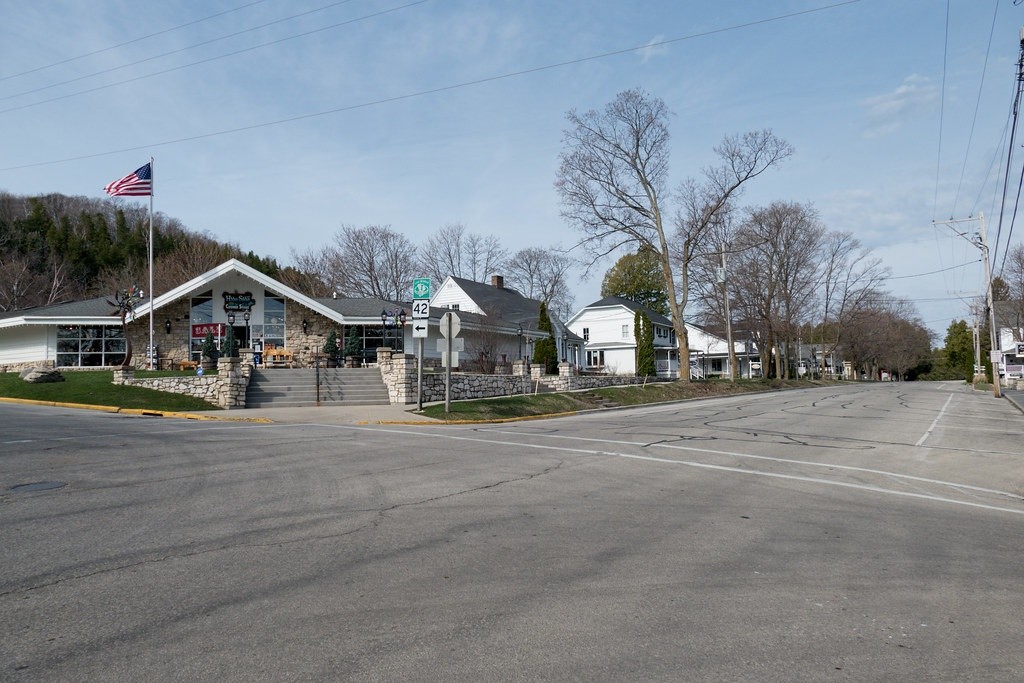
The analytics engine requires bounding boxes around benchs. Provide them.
[261,349,294,369]
[173,361,198,371]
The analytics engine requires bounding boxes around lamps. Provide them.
[164,318,172,334]
[301,319,308,333]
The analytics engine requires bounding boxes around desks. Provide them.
[159,357,173,371]
[351,356,377,368]
[190,297,228,367]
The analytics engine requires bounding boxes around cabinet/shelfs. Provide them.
[249,297,287,367]
[145,344,159,371]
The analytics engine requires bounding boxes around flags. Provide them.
[103,162,151,196]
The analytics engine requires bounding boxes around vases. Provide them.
[479,360,497,374]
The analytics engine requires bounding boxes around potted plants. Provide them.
[201,326,240,370]
[343,326,363,368]
[322,328,340,368]
